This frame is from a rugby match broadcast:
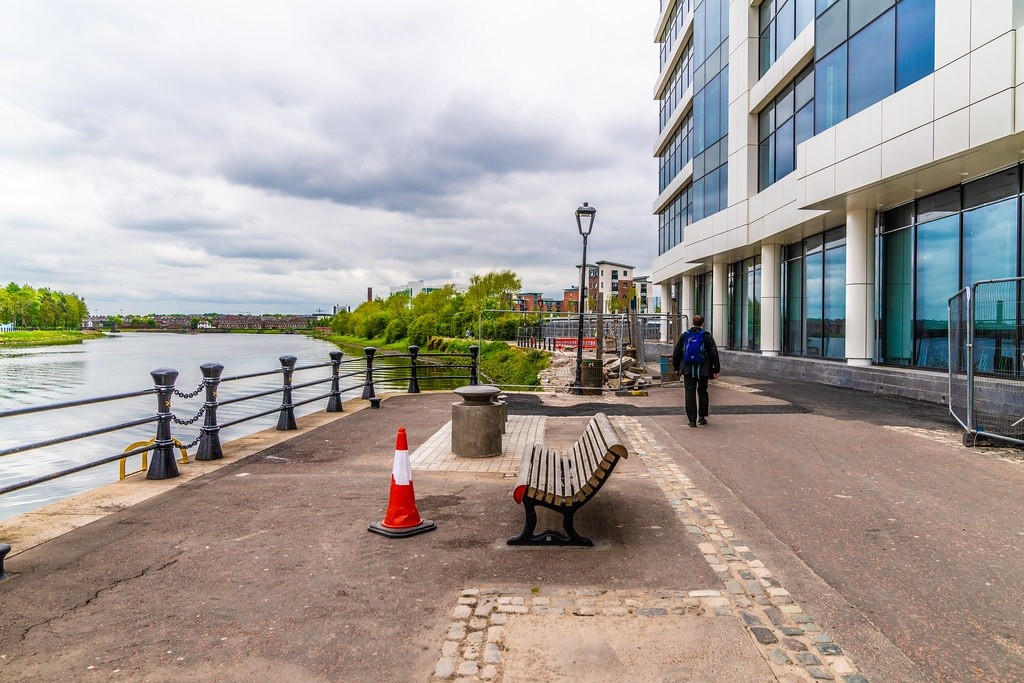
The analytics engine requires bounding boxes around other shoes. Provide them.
[698,418,707,425]
[688,422,696,427]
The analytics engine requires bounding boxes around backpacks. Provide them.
[682,330,708,363]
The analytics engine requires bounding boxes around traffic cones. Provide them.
[368,427,439,539]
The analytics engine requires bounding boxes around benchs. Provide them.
[507,412,630,549]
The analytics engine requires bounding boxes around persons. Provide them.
[465,330,475,339]
[673,314,721,428]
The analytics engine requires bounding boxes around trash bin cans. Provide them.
[581,359,603,395]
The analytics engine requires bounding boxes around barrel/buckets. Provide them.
[660,354,680,382]
[581,359,602,395]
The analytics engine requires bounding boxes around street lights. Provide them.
[571,200,599,394]
[538,297,543,349]
